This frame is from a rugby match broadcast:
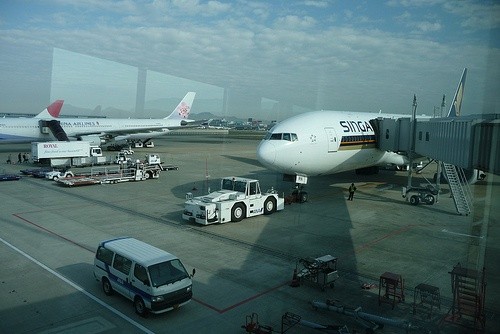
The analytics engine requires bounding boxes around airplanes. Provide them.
[1,90,197,169]
[256,67,485,203]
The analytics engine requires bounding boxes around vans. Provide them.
[92,237,197,316]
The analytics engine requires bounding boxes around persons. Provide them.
[23,152,30,163]
[7,154,12,164]
[348,183,356,200]
[18,152,22,163]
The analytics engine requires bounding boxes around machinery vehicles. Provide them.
[184,175,286,225]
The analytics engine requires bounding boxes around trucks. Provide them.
[31,140,103,166]
